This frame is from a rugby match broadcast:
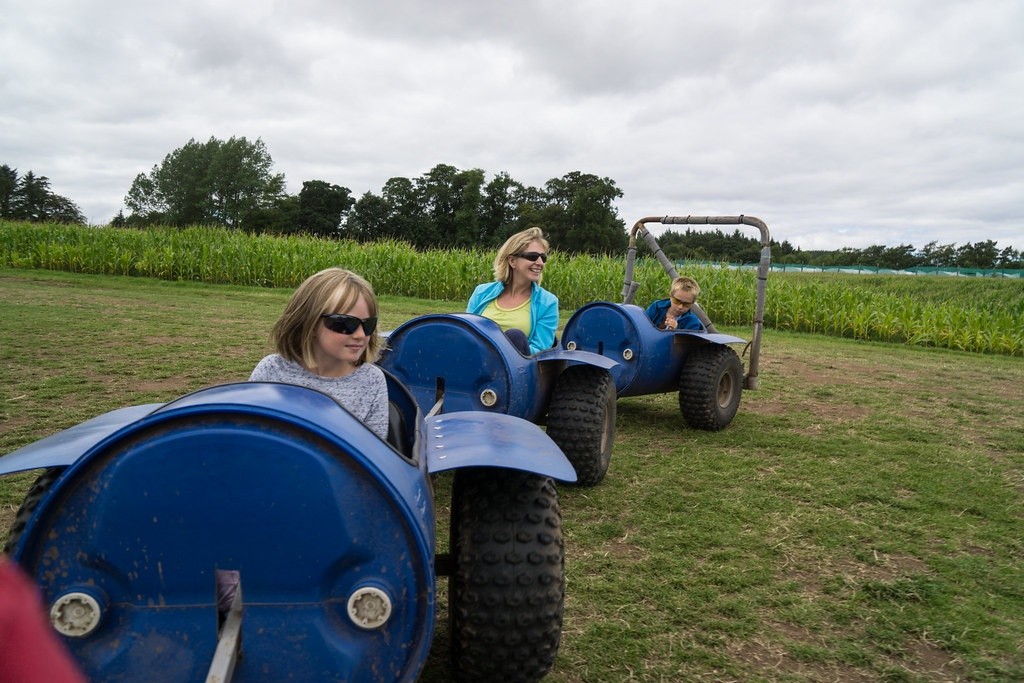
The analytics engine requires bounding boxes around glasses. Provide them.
[516,251,546,263]
[671,295,693,309]
[320,313,378,337]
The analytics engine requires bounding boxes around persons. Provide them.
[248,268,390,441]
[465,227,559,356]
[646,276,702,331]
